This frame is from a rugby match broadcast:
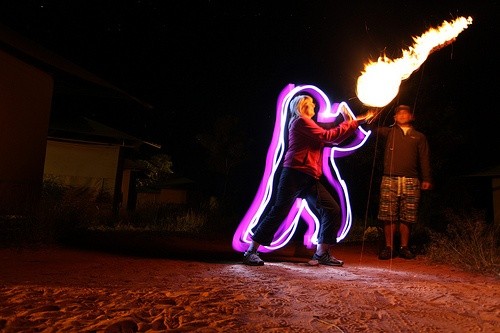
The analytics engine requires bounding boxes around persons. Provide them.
[241,94,374,266]
[366,104,431,260]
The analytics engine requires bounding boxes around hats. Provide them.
[396,104,410,110]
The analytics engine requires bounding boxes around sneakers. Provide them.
[398,245,413,259]
[379,247,394,259]
[243,252,264,265]
[313,250,344,266]
